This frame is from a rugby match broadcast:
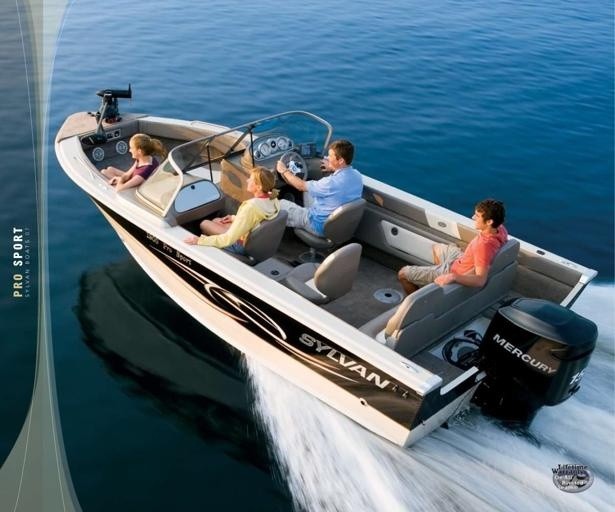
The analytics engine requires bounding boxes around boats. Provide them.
[53,82,600,449]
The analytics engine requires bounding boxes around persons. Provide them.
[397,198,510,296]
[181,166,282,257]
[276,138,365,240]
[101,133,170,194]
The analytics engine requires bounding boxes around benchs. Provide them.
[358,239,520,358]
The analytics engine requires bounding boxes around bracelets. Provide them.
[282,169,289,175]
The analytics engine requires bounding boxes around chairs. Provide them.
[221,198,367,305]
